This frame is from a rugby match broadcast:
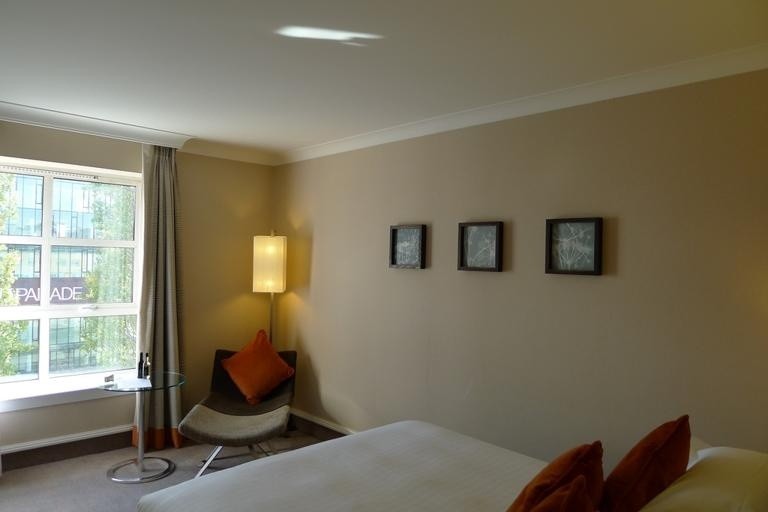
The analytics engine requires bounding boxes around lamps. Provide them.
[250,228,305,442]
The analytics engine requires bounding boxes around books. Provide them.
[104,373,153,392]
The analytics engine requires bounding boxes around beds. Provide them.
[135,415,768,511]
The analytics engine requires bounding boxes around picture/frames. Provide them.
[457,221,504,272]
[543,217,605,277]
[388,223,427,270]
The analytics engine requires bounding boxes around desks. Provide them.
[90,367,191,485]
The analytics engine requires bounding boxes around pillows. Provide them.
[600,411,696,512]
[637,440,768,510]
[218,328,295,406]
[502,436,607,511]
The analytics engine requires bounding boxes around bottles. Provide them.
[137,352,143,379]
[143,353,150,379]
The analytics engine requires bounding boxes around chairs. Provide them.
[175,348,301,481]
